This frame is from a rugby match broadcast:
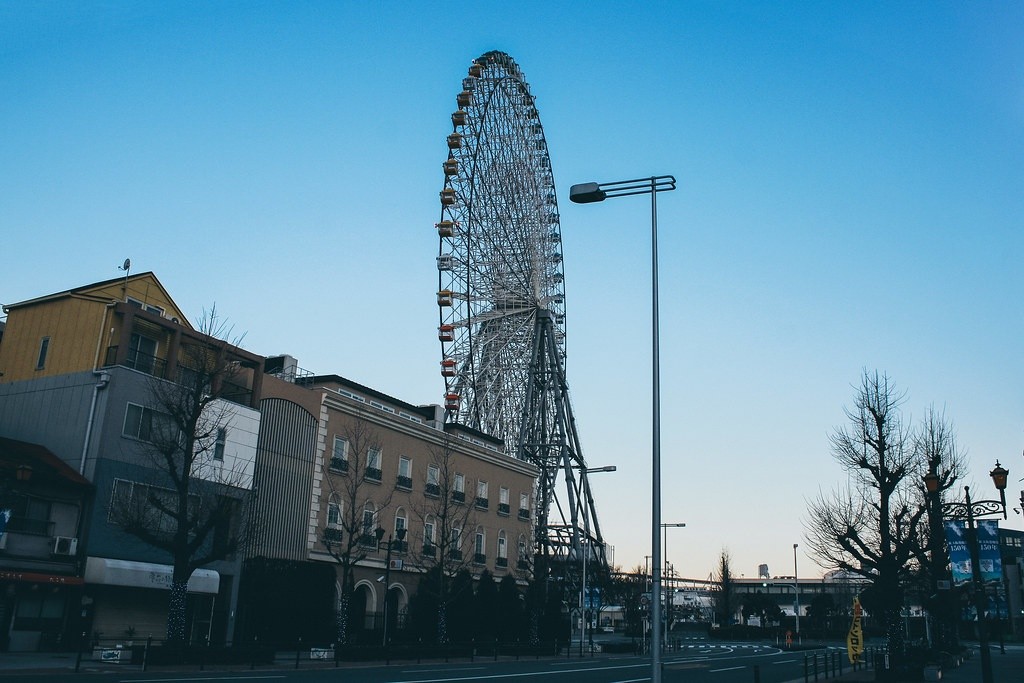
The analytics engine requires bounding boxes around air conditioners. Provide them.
[164,314,181,324]
[51,537,78,556]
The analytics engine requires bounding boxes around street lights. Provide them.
[569,176,676,682]
[794,544,800,635]
[659,523,686,647]
[374,524,408,646]
[579,466,616,657]
[921,458,1009,681]
